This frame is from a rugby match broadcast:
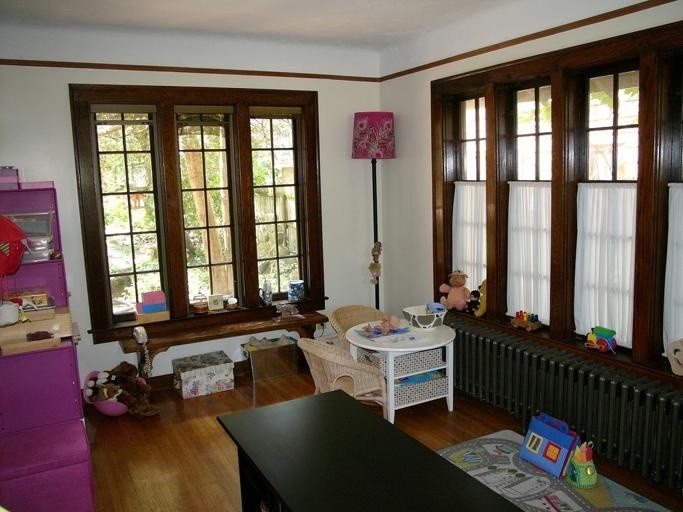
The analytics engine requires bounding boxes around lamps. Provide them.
[350,110,396,310]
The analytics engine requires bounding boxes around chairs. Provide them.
[297,303,388,420]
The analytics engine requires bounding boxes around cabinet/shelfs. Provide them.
[215,389,521,511]
[0,179,95,511]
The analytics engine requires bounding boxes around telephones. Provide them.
[132,326,148,344]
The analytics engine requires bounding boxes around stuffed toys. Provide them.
[474,280,486,317]
[467,290,481,313]
[86,360,158,418]
[244,334,290,353]
[440,269,471,311]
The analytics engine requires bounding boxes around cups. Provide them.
[288,279,304,304]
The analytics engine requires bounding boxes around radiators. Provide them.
[446,320,683,490]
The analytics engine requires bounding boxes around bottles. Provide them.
[194,292,208,315]
[261,281,272,308]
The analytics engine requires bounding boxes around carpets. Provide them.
[437,428,669,511]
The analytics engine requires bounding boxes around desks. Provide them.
[345,318,456,426]
[119,312,328,418]
